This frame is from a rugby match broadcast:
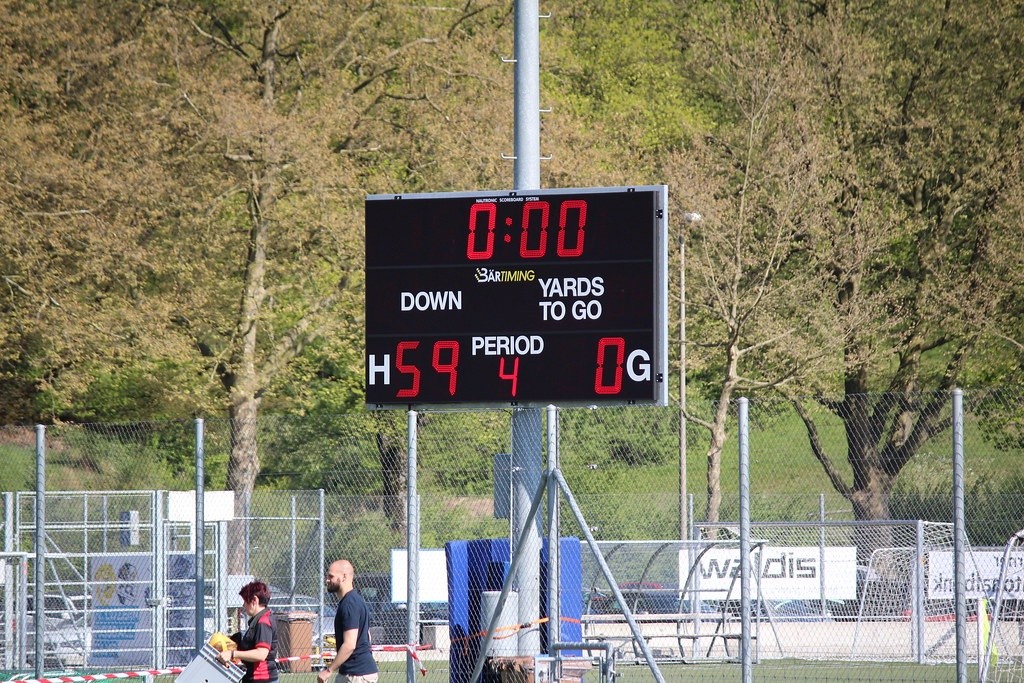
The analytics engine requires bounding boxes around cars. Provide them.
[583,563,910,622]
[0,567,450,666]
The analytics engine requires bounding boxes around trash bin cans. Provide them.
[274,611,317,673]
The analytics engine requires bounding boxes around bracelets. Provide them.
[327,667,332,673]
[231,650,234,660]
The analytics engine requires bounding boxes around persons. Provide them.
[216,581,279,683]
[316,560,379,683]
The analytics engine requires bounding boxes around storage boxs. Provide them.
[175,643,247,683]
